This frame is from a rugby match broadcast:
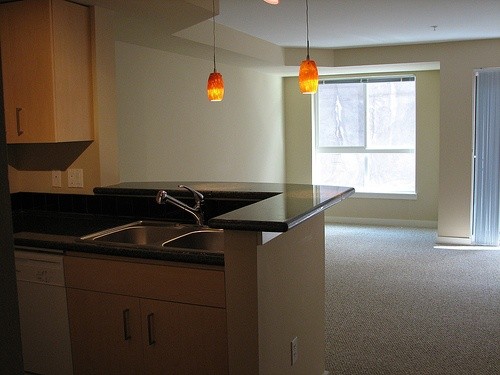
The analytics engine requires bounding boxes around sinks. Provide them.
[160,227,226,255]
[79,220,190,247]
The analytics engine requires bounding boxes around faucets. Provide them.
[154,182,207,224]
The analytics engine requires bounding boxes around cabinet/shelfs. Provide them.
[0,0,119,194]
[63,253,229,375]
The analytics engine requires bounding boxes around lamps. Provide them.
[205,0,226,102]
[298,0,319,95]
